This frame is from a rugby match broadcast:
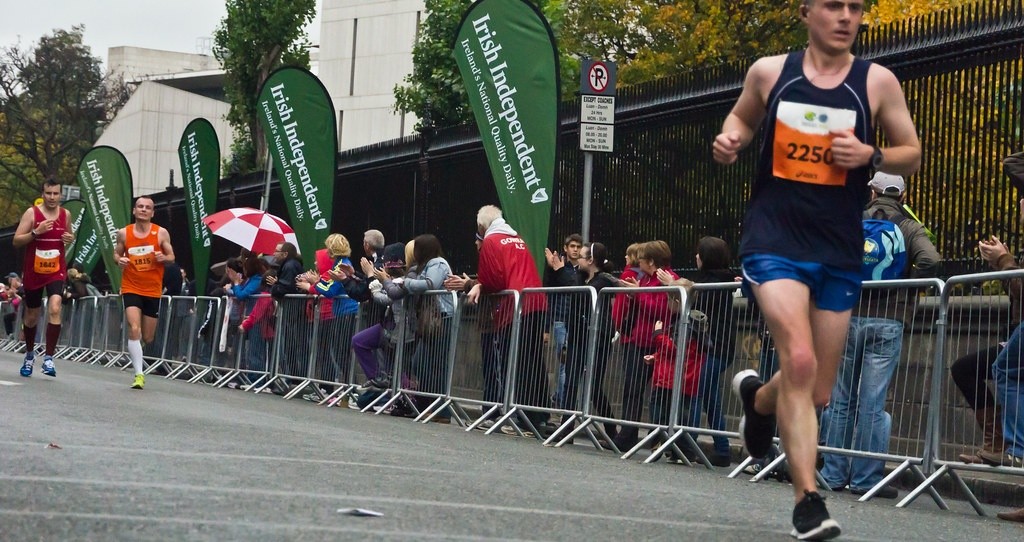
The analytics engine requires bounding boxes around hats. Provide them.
[382,242,406,269]
[4,272,18,278]
[867,171,906,197]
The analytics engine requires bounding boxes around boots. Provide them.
[958,406,1008,464]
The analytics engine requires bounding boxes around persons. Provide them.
[443,231,500,430]
[0,272,25,339]
[712,0,922,542]
[111,196,175,388]
[143,230,458,424]
[741,275,780,382]
[467,205,550,438]
[818,172,940,498]
[13,176,75,376]
[543,234,739,467]
[62,268,92,347]
[950,150,1024,522]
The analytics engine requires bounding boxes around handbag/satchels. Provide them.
[417,278,445,339]
[11,297,21,306]
[230,300,243,326]
[378,304,396,332]
[558,345,570,365]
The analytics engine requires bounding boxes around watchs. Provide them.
[868,147,883,168]
[31,230,37,237]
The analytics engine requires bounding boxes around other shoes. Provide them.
[742,463,770,478]
[851,483,898,498]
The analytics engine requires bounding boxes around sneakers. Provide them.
[227,378,732,468]
[732,369,777,461]
[19,356,36,377]
[976,448,1023,467]
[130,374,146,389]
[40,355,56,375]
[790,491,842,542]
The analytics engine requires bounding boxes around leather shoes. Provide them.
[997,508,1024,522]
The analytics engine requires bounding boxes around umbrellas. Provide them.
[202,207,301,255]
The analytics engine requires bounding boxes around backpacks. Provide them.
[86,283,102,306]
[859,209,908,302]
